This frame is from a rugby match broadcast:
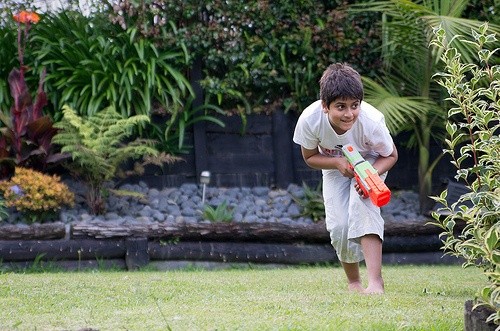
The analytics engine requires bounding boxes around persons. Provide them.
[292,63,399,295]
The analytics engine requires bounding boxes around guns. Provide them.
[342,143,392,208]
